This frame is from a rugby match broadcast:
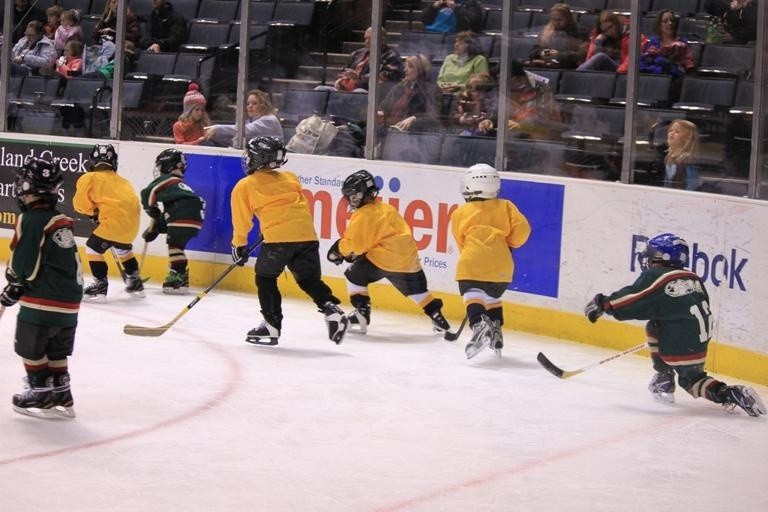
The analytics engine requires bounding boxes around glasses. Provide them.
[24,32,36,38]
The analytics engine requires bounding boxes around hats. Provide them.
[181,83,207,116]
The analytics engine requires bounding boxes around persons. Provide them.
[704,1,759,46]
[362,31,560,143]
[141,147,206,290]
[450,162,532,356]
[173,83,216,145]
[0,158,84,409]
[584,233,767,417]
[230,135,349,345]
[422,0,483,33]
[528,3,695,83]
[664,119,701,189]
[326,170,450,333]
[204,89,286,148]
[0,0,187,80]
[72,143,144,295]
[314,25,402,94]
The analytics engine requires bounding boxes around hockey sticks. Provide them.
[444,315,468,341]
[536,343,648,379]
[108,246,150,285]
[124,234,264,337]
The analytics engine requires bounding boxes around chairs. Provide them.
[0,0,768,202]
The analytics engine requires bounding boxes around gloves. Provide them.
[327,239,345,265]
[232,247,249,268]
[0,284,25,307]
[143,224,160,242]
[146,204,161,219]
[585,293,605,323]
[88,214,99,225]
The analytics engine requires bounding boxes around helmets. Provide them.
[239,135,289,175]
[151,147,188,178]
[14,160,62,210]
[459,163,501,202]
[637,232,690,271]
[89,144,118,171]
[341,170,379,207]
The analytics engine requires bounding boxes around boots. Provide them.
[648,370,675,393]
[162,268,189,288]
[83,276,108,294]
[124,270,144,293]
[348,300,371,325]
[247,309,282,338]
[718,385,756,417]
[22,371,74,408]
[12,375,55,409]
[317,302,344,339]
[429,310,503,359]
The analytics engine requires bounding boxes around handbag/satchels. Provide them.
[426,4,458,34]
[285,114,340,153]
